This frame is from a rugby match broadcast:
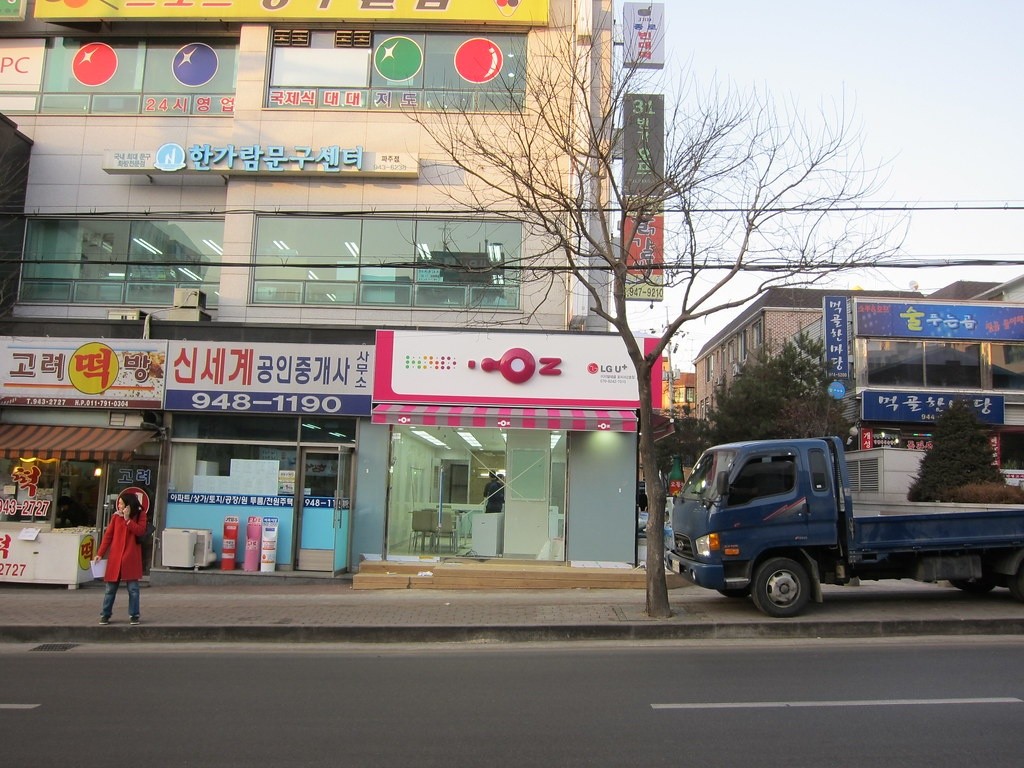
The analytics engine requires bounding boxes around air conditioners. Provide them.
[161,526,213,571]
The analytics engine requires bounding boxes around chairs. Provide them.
[408,508,457,553]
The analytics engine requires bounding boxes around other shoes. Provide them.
[99,615,108,624]
[129,616,139,624]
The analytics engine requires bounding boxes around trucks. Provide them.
[667,433,1024,612]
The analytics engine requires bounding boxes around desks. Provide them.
[408,510,461,548]
[1,530,98,590]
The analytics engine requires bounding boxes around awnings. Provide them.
[0,423,159,463]
[371,405,638,432]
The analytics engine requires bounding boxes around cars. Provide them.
[639,481,645,495]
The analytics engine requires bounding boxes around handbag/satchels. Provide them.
[135,509,157,545]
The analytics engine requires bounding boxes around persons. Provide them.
[638,489,648,512]
[483,470,505,513]
[94,493,146,624]
